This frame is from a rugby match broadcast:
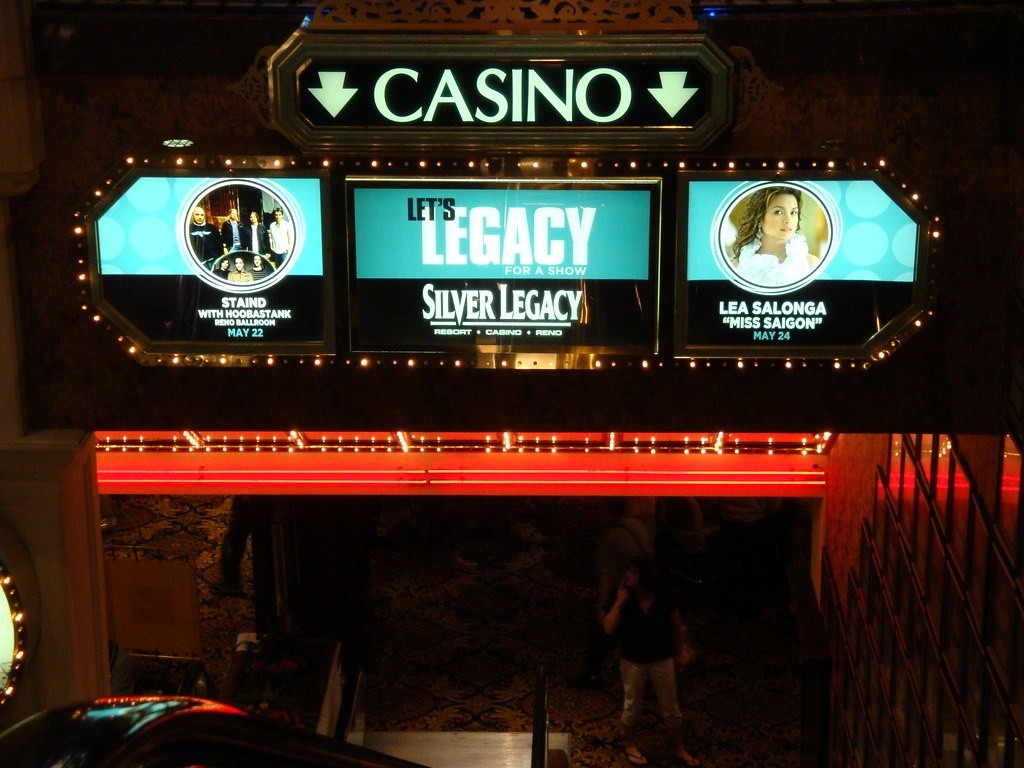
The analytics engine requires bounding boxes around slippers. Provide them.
[677,752,701,767]
[626,752,648,766]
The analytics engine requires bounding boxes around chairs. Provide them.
[335,664,572,768]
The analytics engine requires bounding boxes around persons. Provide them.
[191,206,292,283]
[729,186,818,287]
[581,499,653,675]
[603,555,702,768]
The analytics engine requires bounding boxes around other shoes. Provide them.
[567,671,601,687]
[224,591,253,609]
